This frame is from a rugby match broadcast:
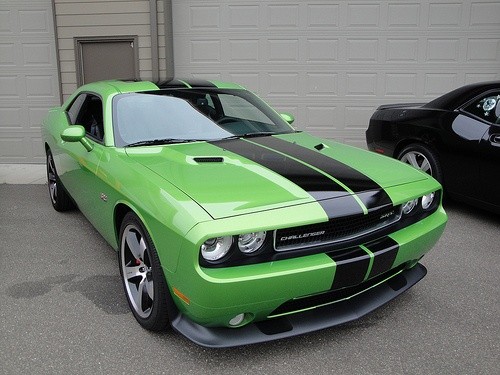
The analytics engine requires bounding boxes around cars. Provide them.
[41,77,445,347]
[365,82,500,215]
